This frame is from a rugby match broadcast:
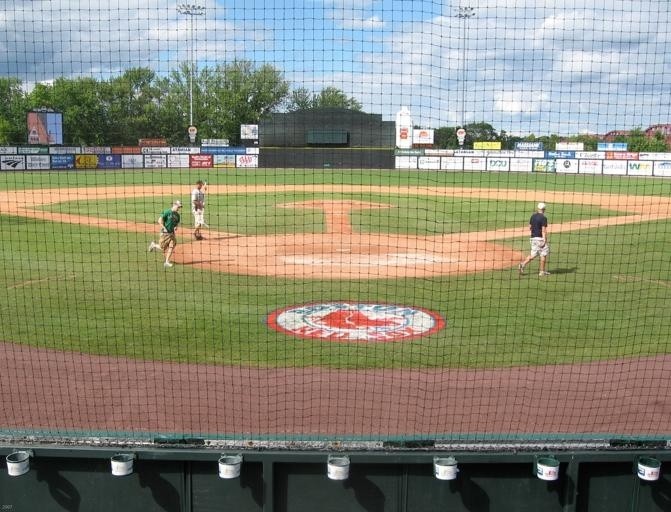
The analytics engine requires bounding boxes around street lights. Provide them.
[176,4,206,126]
[454,7,475,129]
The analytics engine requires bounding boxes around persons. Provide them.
[518,201,553,276]
[192,180,206,241]
[149,200,184,267]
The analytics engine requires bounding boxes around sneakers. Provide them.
[518,263,550,277]
[164,263,173,267]
[149,241,156,252]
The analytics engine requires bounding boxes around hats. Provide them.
[538,203,546,210]
